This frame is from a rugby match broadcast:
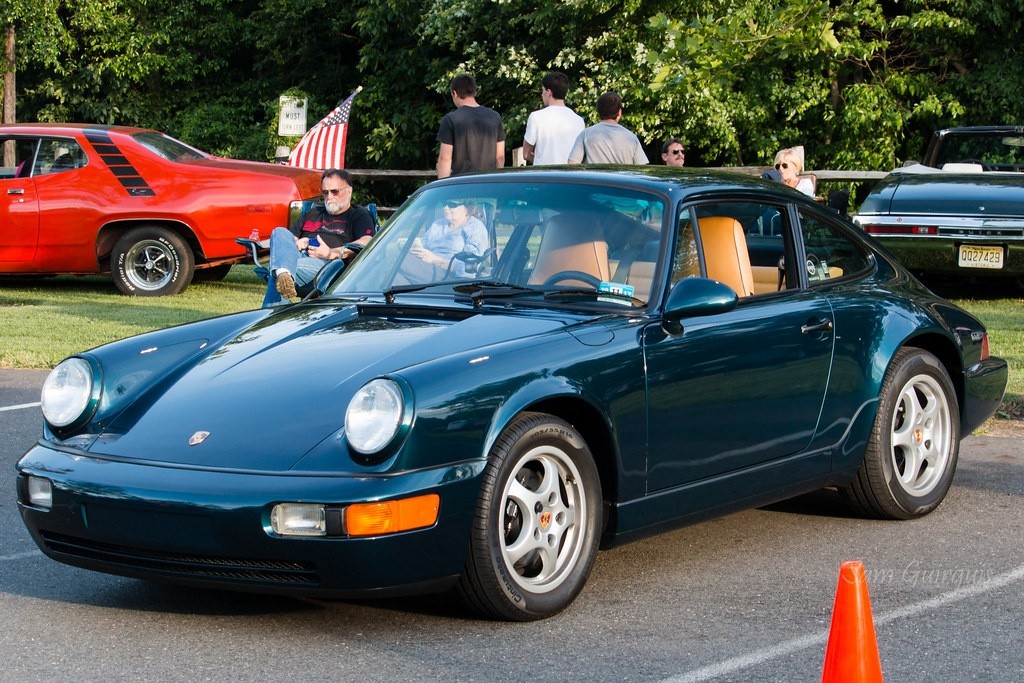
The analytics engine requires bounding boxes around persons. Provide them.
[354,196,491,292]
[262,169,379,307]
[436,74,506,179]
[772,149,814,198]
[568,92,650,165]
[661,139,685,167]
[523,72,585,164]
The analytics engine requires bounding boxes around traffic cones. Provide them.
[819,560,886,683]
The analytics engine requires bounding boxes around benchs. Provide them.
[609,248,787,300]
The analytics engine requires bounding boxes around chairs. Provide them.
[16,154,42,177]
[527,213,610,287]
[50,153,74,174]
[673,218,754,297]
[425,198,498,279]
[234,200,377,284]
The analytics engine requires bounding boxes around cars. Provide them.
[15,173,1013,624]
[851,122,1023,290]
[0,123,328,297]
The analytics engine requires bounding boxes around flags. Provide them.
[283,97,353,170]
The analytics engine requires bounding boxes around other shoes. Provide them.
[275,273,298,300]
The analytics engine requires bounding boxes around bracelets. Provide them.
[439,257,445,268]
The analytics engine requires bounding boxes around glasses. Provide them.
[668,149,687,155]
[774,163,793,171]
[321,186,348,197]
